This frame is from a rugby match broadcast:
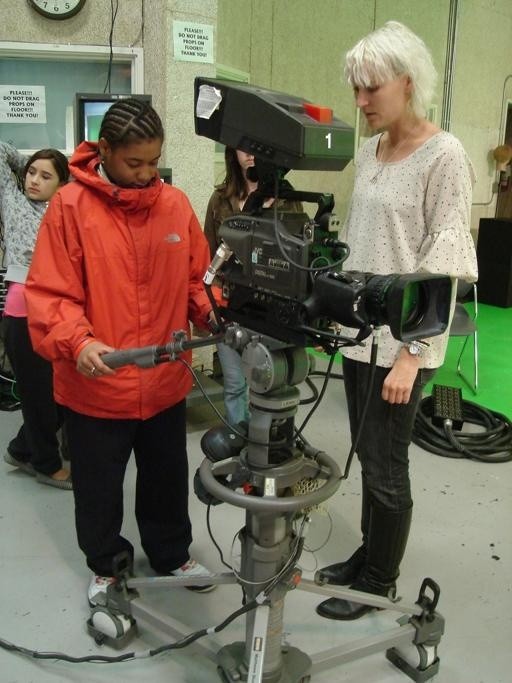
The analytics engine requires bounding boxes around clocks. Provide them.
[24,0,86,23]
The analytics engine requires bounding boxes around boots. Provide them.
[316,497,369,587]
[317,499,414,619]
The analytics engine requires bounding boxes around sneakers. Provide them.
[3,449,35,475]
[88,572,118,608]
[167,558,217,593]
[36,471,73,489]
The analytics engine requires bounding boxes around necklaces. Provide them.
[368,121,418,184]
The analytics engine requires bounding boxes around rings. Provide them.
[89,366,96,377]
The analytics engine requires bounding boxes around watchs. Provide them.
[401,342,425,359]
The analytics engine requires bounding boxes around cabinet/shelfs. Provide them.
[474,213,512,308]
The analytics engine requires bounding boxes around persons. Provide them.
[0,141,73,490]
[316,20,479,620]
[24,99,230,607]
[203,143,303,425]
